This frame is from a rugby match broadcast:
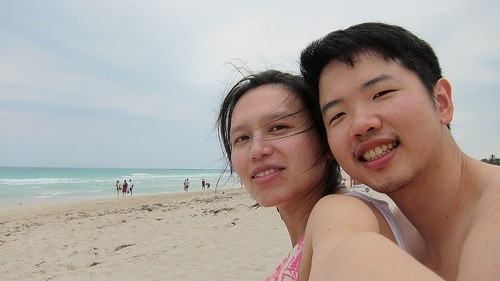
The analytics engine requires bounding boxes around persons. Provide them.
[116,179,134,197]
[214,69,407,281]
[300,22,500,281]
[202,178,206,190]
[183,179,189,191]
[206,183,210,190]
